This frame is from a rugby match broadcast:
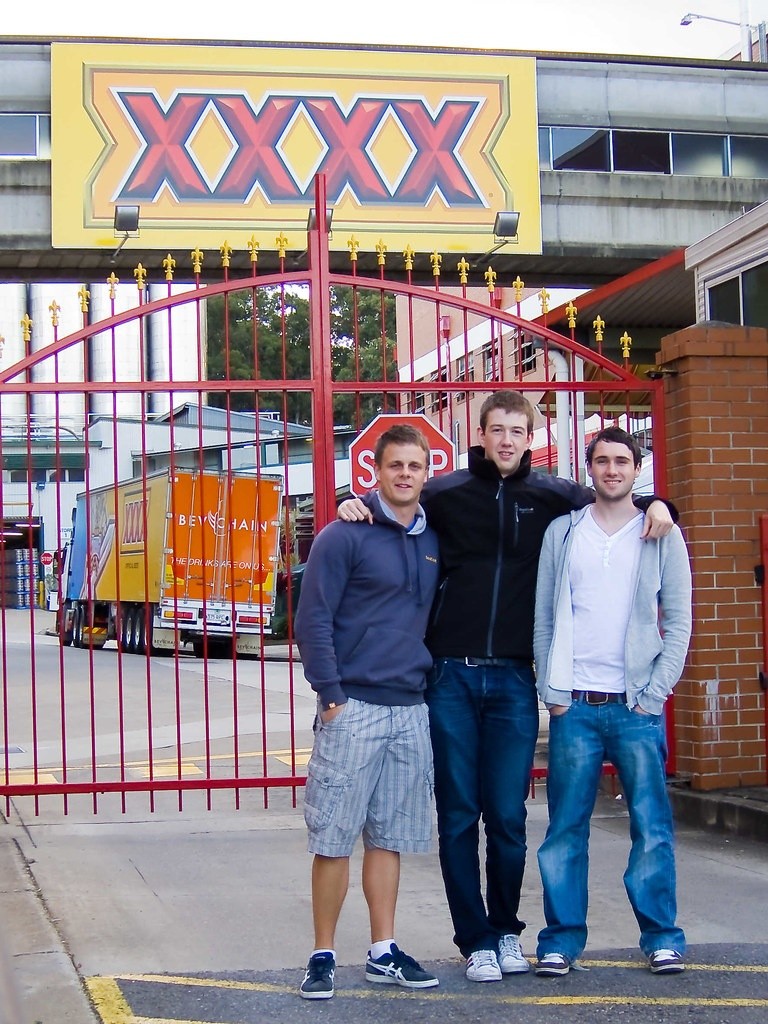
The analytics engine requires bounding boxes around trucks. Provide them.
[55,465,284,660]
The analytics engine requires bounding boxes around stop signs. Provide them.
[41,553,54,566]
[348,415,455,499]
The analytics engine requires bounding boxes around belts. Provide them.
[572,691,627,705]
[451,657,514,667]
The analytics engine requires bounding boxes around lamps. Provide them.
[295,208,334,261]
[474,212,521,264]
[113,205,140,260]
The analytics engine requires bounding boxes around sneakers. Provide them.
[498,934,529,973]
[365,943,439,988]
[648,949,685,972]
[300,952,337,998]
[534,952,575,975]
[465,950,502,981]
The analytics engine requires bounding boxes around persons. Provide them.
[337,390,678,982]
[294,425,439,998]
[534,427,692,975]
